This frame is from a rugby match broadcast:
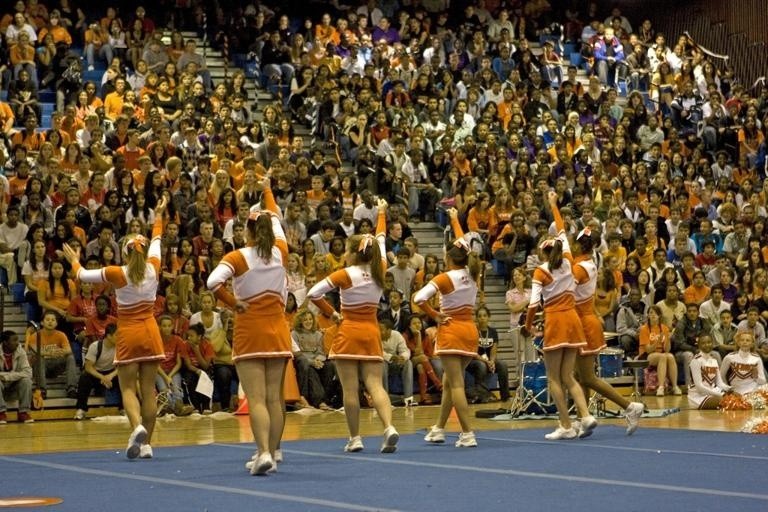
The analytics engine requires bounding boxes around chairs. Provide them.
[14,283,35,328]
[0,45,125,128]
[556,43,581,68]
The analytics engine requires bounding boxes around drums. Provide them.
[595,346,625,378]
[519,359,557,415]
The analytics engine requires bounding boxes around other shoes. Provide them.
[319,403,329,409]
[422,392,432,404]
[471,395,483,403]
[404,396,418,406]
[223,407,232,413]
[176,402,194,416]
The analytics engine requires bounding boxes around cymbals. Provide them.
[523,310,544,316]
[602,331,624,340]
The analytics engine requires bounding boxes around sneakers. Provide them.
[138,443,154,459]
[654,384,665,396]
[543,426,577,441]
[671,385,682,396]
[243,447,285,476]
[379,425,400,453]
[0,411,7,425]
[38,388,48,398]
[455,430,478,448]
[124,424,148,460]
[570,416,597,439]
[341,434,364,453]
[422,424,446,443]
[72,408,85,420]
[65,388,78,398]
[16,411,35,425]
[622,401,645,435]
